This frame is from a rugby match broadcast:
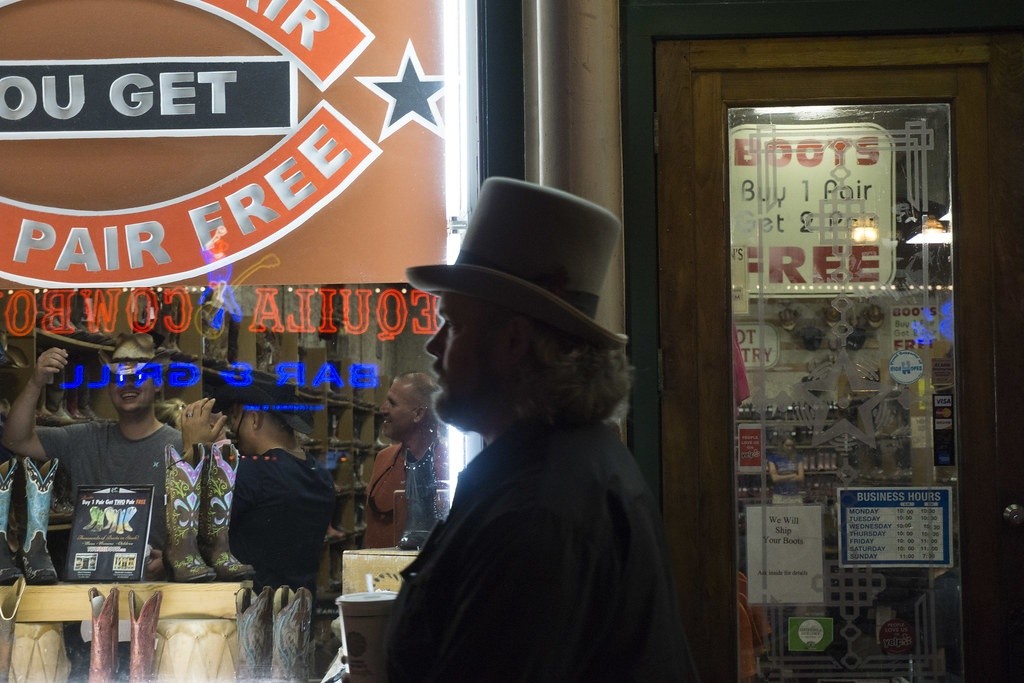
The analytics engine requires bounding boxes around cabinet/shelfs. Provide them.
[34,283,405,680]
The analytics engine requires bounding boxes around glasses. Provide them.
[226,404,249,448]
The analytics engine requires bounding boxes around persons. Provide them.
[368,176,699,683]
[177,381,346,639]
[1,331,189,583]
[363,371,455,549]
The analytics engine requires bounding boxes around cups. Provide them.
[337,592,400,683]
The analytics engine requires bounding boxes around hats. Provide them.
[411,176,628,349]
[97,333,171,375]
[224,368,315,436]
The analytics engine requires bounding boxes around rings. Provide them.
[187,412,192,417]
